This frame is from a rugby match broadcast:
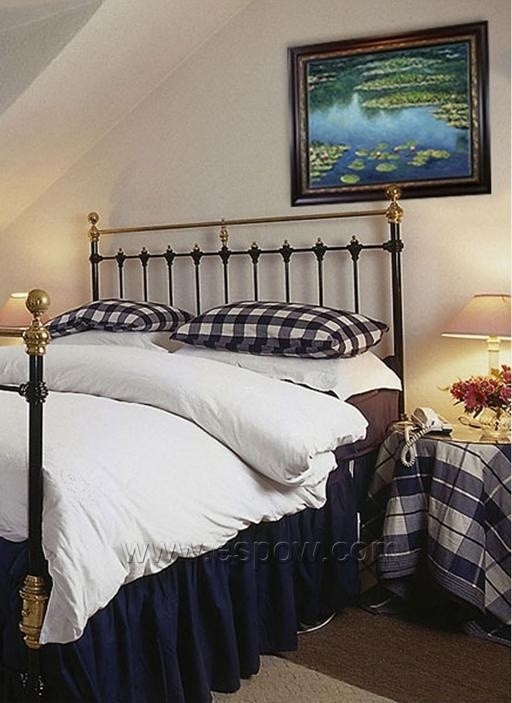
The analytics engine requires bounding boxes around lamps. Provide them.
[441,293,512,433]
[0,292,50,327]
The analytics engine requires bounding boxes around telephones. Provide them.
[411,406,454,435]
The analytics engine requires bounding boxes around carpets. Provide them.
[211,655,397,703]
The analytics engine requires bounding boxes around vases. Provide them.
[480,407,512,439]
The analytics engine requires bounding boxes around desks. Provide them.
[356,419,512,649]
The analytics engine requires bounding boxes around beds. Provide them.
[0,184,407,703]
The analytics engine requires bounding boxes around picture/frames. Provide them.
[287,19,491,207]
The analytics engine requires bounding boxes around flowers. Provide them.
[437,364,512,420]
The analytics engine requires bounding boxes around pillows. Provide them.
[42,298,194,339]
[49,329,180,353]
[172,346,401,402]
[168,301,389,359]
